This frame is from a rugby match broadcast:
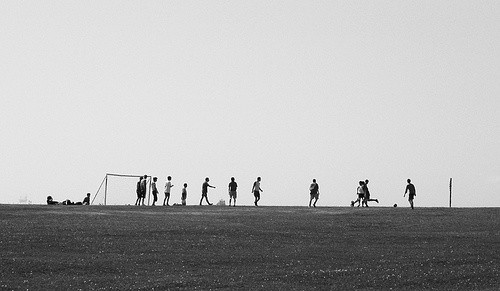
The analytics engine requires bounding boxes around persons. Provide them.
[180,183,187,205]
[140,174,147,206]
[83,192,91,205]
[163,175,174,206]
[228,176,238,206]
[403,178,416,209]
[46,196,66,205]
[360,181,379,207]
[199,177,216,206]
[308,178,320,208]
[357,181,364,208]
[251,176,263,207]
[354,179,369,207]
[152,176,159,206]
[134,176,143,206]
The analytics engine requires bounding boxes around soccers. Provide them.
[393,204,397,208]
